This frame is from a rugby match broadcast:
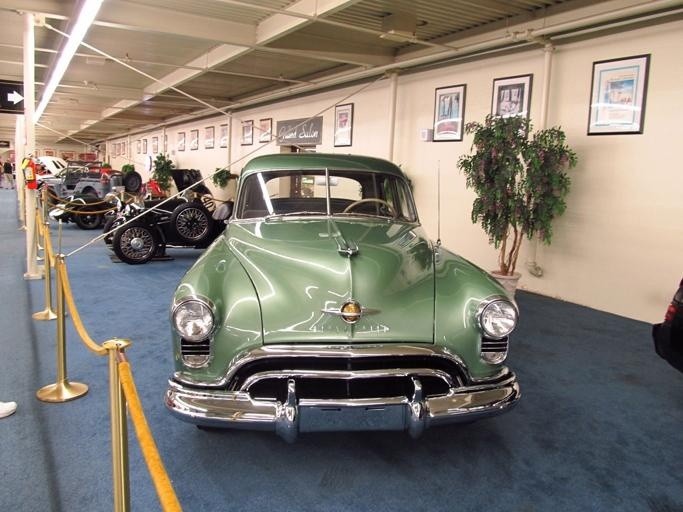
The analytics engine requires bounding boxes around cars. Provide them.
[164,153,523,444]
[651,277,683,374]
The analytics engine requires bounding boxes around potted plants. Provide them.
[456,113,577,301]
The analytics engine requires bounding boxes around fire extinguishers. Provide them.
[22,153,37,189]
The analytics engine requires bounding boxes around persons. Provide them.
[1,157,14,190]
[0,161,3,188]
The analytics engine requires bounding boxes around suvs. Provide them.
[43,159,233,265]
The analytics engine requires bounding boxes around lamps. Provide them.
[379,13,418,44]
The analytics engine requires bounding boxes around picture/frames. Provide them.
[333,103,354,147]
[10,117,273,161]
[586,53,652,135]
[432,83,467,143]
[489,73,534,140]
